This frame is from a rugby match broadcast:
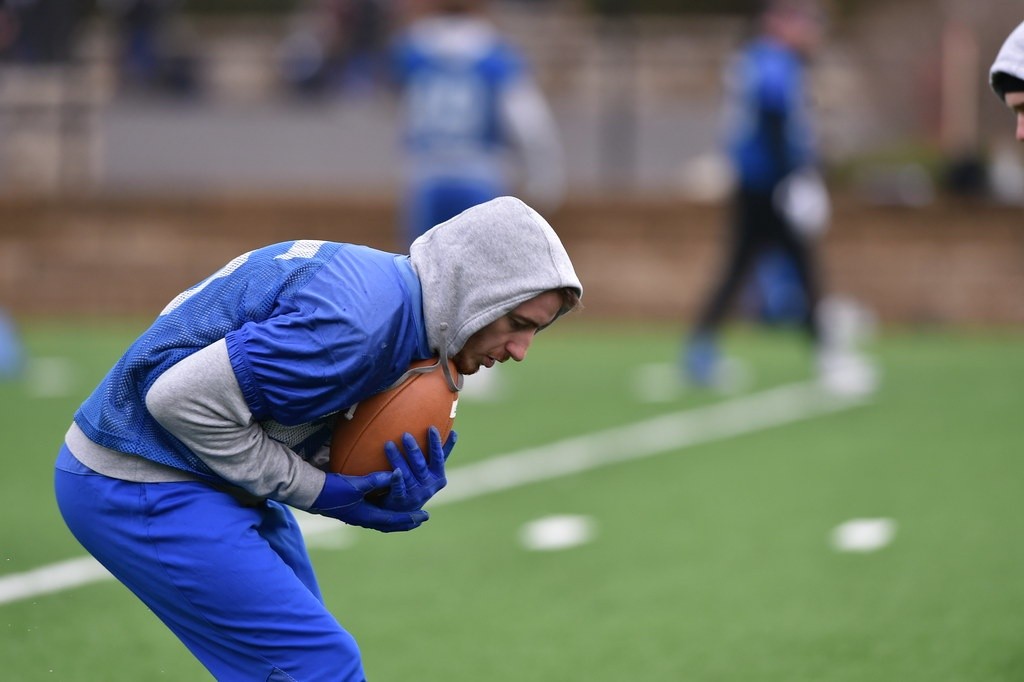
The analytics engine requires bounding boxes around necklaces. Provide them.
[53,195,586,682]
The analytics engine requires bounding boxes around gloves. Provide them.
[384,425,458,512]
[306,470,429,533]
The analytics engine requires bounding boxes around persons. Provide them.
[0,0,1024,406]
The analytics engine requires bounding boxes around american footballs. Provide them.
[329,357,461,477]
